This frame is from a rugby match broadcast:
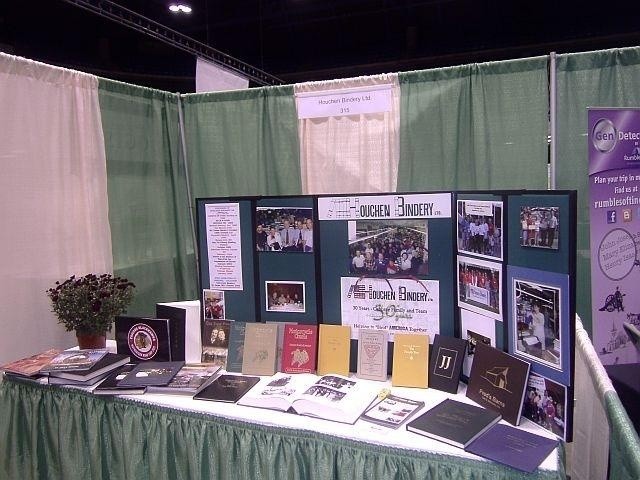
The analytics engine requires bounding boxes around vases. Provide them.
[77,326,106,348]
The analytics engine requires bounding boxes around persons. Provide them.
[520,209,559,248]
[268,292,300,308]
[458,213,505,261]
[347,224,429,276]
[255,207,314,253]
[459,264,500,313]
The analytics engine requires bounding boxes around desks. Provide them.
[0,340,566,480]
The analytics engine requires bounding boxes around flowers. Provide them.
[46,274,136,332]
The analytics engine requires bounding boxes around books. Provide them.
[1,297,561,472]
